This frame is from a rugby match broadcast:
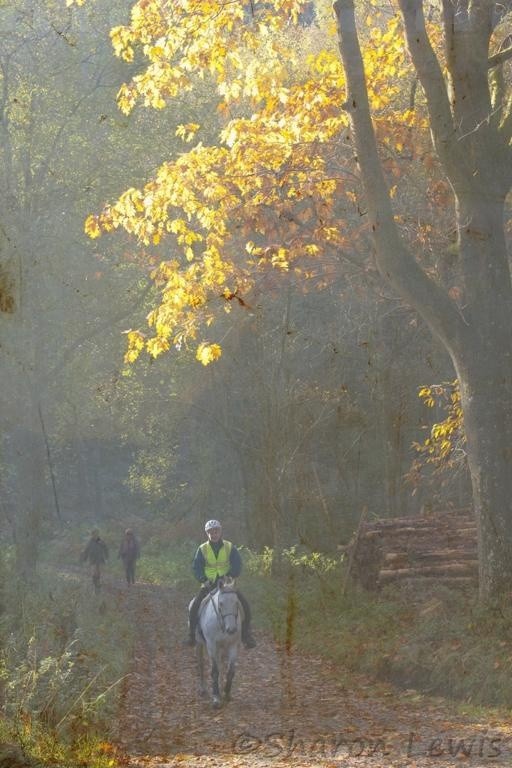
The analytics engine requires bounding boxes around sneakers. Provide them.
[242,635,256,648]
[181,637,195,647]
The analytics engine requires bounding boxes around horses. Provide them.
[187,576,245,707]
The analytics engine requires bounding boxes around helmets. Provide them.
[204,519,221,532]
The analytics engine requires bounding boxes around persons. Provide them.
[184,520,256,649]
[80,530,108,585]
[116,529,139,584]
